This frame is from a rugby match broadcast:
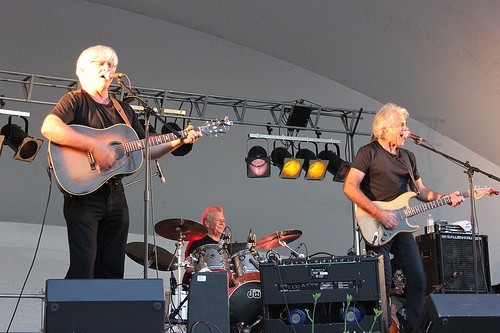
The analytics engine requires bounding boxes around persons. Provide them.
[184,206,236,286]
[41,45,203,278]
[343,104,466,333]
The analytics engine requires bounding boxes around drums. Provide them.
[229,248,260,282]
[185,244,232,283]
[229,280,264,333]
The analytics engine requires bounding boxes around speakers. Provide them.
[187,272,230,333]
[414,232,500,333]
[45,279,166,333]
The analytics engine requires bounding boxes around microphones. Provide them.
[404,131,426,142]
[289,244,303,260]
[246,230,254,249]
[453,272,463,276]
[105,71,126,80]
[219,228,226,245]
[171,271,177,286]
[155,160,165,183]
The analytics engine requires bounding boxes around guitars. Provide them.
[355,185,499,248]
[47,115,233,197]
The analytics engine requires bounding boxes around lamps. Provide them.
[245,137,270,178]
[296,141,329,181]
[139,119,157,135]
[271,139,305,180]
[162,117,193,156]
[318,143,351,182]
[0,115,43,162]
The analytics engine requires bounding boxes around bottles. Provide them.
[426,213,434,233]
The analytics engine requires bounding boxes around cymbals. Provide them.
[124,242,179,272]
[155,218,208,242]
[255,229,302,250]
